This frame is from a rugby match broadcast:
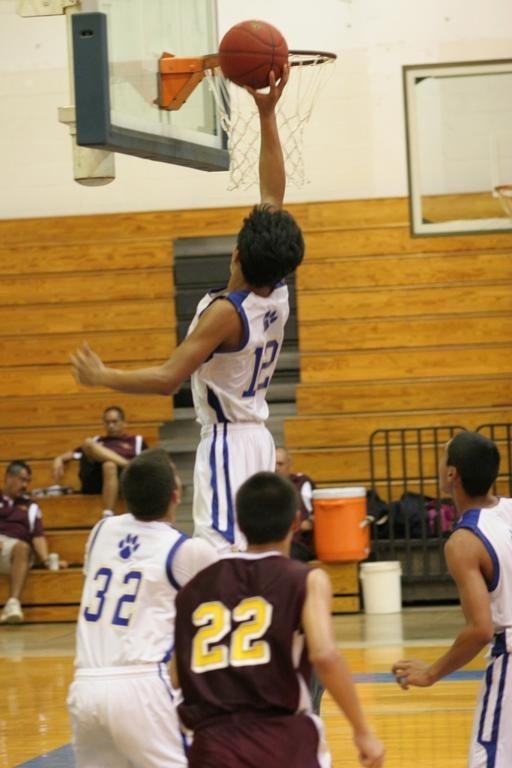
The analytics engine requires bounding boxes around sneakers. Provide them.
[1,602,25,623]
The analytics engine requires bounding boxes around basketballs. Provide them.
[220,20,287,88]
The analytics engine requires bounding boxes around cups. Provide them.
[47,552,60,571]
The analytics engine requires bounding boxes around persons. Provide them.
[52,406,149,518]
[0,460,68,624]
[172,471,385,768]
[70,61,304,553]
[67,446,219,768]
[275,446,316,560]
[391,430,512,768]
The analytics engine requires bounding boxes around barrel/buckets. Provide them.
[360,561,403,614]
[311,487,371,563]
